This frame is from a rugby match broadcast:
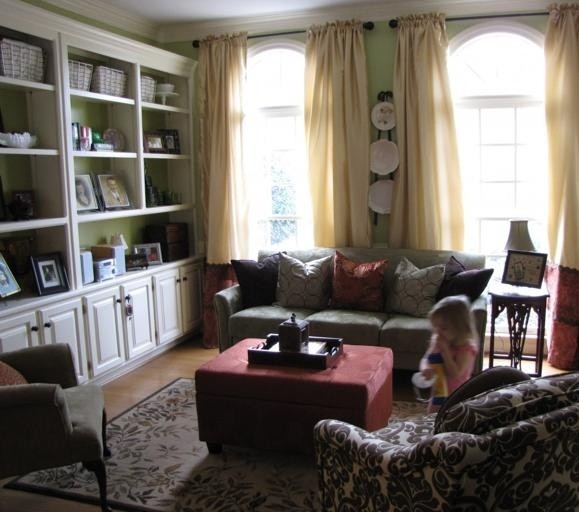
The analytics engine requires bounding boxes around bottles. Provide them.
[72,122,93,152]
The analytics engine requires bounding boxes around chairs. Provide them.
[0,343,115,512]
[312,402,579,512]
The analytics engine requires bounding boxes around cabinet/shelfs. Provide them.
[0,294,89,387]
[153,255,206,356]
[1,1,200,292]
[83,273,158,382]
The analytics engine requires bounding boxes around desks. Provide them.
[488,290,550,377]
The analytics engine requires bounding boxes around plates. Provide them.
[369,140,400,175]
[367,179,396,214]
[370,102,397,131]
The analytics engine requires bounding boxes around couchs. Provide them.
[213,248,487,372]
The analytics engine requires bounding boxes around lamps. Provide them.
[503,221,535,251]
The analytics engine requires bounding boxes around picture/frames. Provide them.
[30,252,70,296]
[133,243,163,265]
[13,190,37,219]
[74,171,134,214]
[501,249,548,289]
[143,129,180,154]
[0,252,21,298]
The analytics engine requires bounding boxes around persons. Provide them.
[422,296,479,415]
[75,178,93,208]
[104,176,126,206]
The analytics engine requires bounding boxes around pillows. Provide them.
[436,256,495,304]
[230,251,287,306]
[0,360,29,386]
[273,251,333,309]
[435,366,530,425]
[330,250,389,312]
[433,369,579,434]
[385,257,445,319]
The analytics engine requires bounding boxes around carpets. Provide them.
[3,376,428,512]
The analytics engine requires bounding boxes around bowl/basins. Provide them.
[1,130,36,148]
